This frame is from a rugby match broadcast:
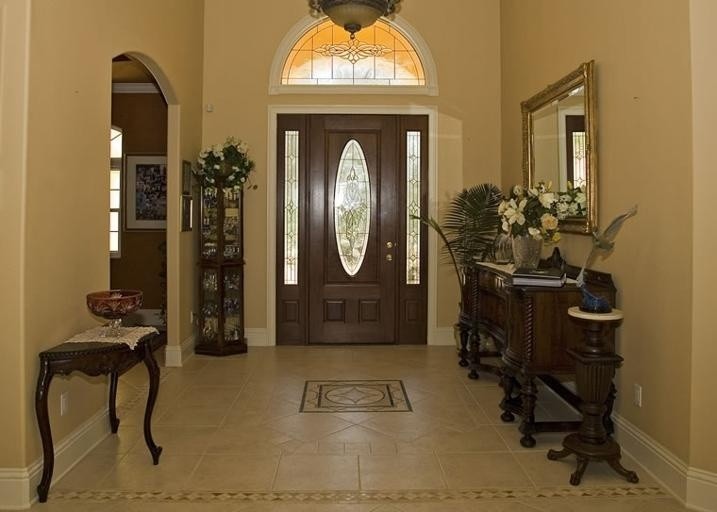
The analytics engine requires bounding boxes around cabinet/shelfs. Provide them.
[194,174,247,356]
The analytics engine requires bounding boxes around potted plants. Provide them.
[407,183,508,357]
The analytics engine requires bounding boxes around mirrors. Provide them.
[519,60,597,236]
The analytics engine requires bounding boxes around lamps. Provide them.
[308,0,402,40]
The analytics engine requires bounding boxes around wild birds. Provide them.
[576,203,638,288]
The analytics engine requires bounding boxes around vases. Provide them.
[511,234,543,273]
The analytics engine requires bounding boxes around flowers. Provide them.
[497,179,565,245]
[190,135,262,190]
[556,180,586,218]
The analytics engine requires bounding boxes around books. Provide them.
[511,272,566,288]
[510,267,565,280]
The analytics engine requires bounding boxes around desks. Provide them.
[456,246,617,448]
[34,323,167,502]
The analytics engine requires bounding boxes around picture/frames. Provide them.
[122,151,167,234]
[179,160,194,232]
